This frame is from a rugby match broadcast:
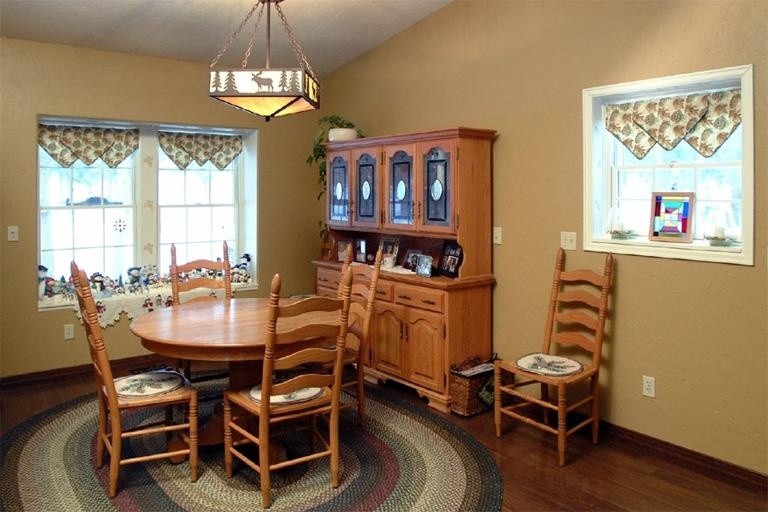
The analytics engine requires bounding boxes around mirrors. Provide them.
[354,237,369,264]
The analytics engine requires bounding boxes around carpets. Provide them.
[0,369,504,512]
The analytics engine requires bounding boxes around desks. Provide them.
[129,297,357,469]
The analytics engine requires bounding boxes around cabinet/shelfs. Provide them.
[383,126,497,235]
[323,136,386,230]
[310,261,377,386]
[373,272,497,414]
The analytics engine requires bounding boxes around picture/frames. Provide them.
[437,239,464,278]
[402,249,423,272]
[416,254,433,277]
[379,236,400,267]
[336,239,354,263]
[648,191,695,243]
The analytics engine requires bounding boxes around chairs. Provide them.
[170,241,232,418]
[223,267,354,509]
[300,240,383,429]
[70,260,199,498]
[493,247,614,467]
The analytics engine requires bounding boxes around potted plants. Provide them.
[304,111,367,238]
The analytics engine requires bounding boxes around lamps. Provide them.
[207,0,321,123]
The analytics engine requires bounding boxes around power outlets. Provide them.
[641,375,656,399]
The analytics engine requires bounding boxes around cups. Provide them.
[383,253,397,269]
